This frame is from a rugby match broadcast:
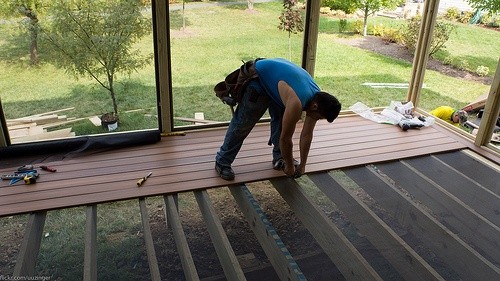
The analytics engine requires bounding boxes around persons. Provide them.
[214,57,342,181]
[428,106,468,128]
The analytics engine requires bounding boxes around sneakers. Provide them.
[272,158,299,168]
[215,162,235,181]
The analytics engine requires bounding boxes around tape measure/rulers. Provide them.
[24,174,36,184]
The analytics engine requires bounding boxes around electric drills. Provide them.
[399,118,424,131]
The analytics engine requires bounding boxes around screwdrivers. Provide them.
[137,172,152,186]
